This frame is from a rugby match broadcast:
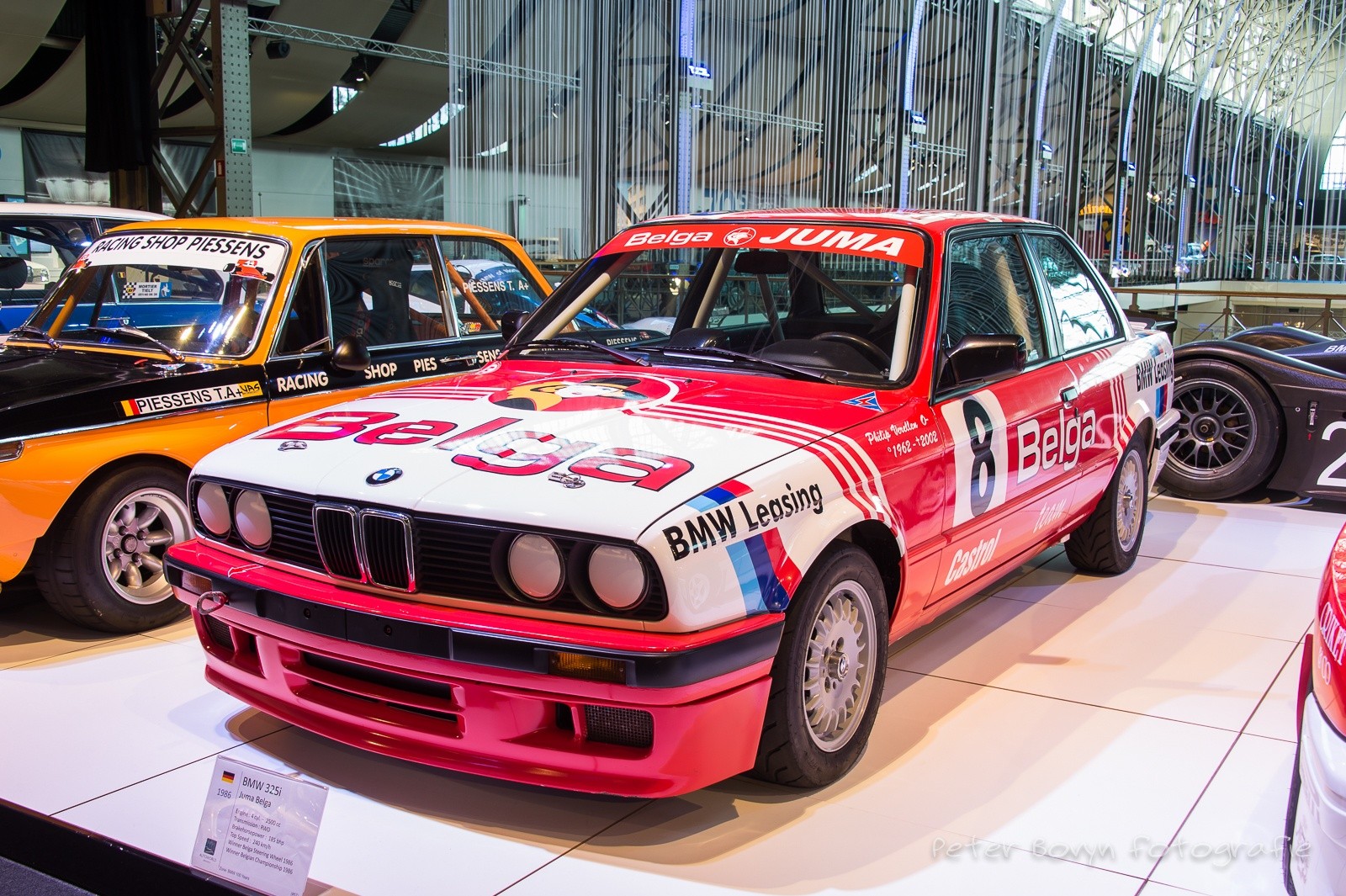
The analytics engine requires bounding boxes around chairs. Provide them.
[0,255,28,305]
[281,250,364,357]
[867,261,994,357]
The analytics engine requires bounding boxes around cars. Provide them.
[155,203,1181,798]
[3,214,596,638]
[0,196,280,332]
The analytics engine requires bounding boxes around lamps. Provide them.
[653,69,1180,212]
[153,22,566,122]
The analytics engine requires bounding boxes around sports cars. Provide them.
[1153,323,1345,504]
